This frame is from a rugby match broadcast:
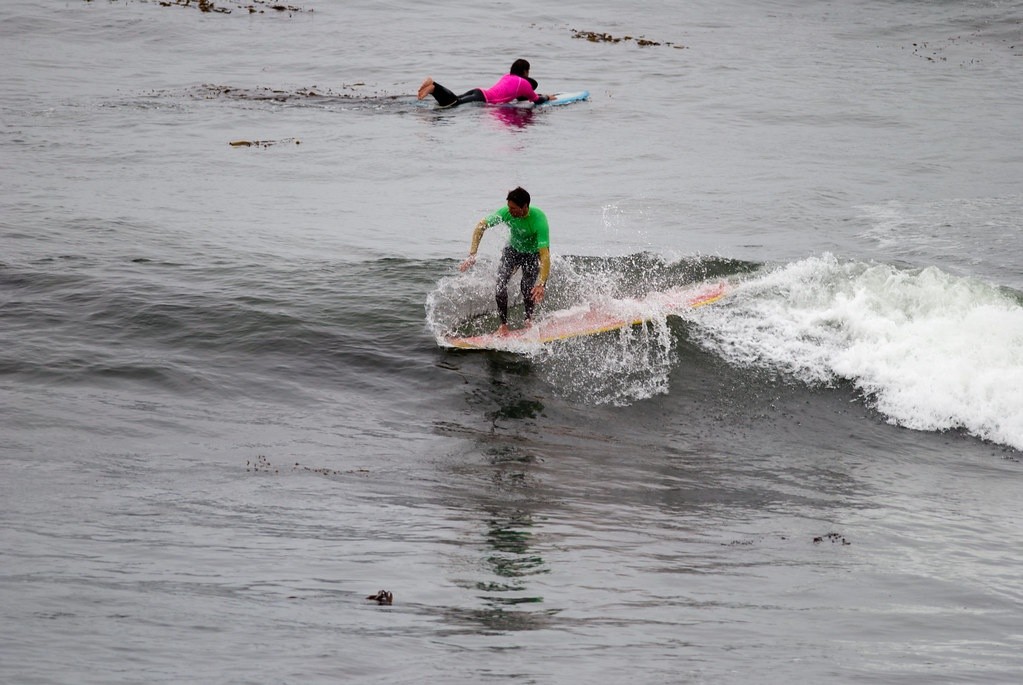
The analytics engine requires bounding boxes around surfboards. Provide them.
[447,282,725,351]
[471,90,589,107]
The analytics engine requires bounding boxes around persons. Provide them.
[460,187,550,335]
[418,58,557,108]
